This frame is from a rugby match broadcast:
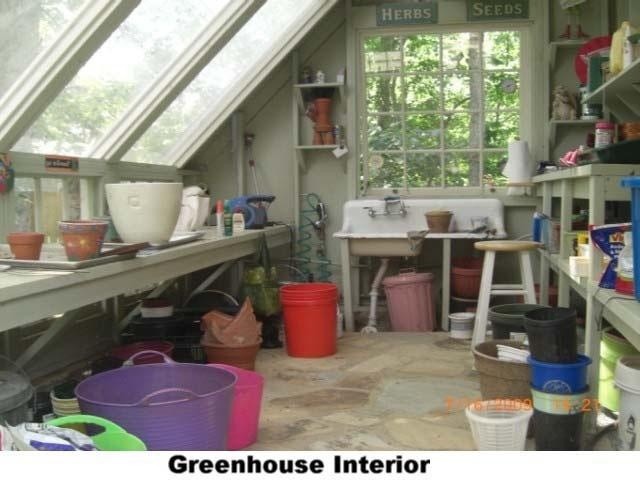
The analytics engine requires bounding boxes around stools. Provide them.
[472,239,541,352]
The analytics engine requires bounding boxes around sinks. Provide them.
[331,229,422,257]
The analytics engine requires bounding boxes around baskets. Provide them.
[464,398,535,450]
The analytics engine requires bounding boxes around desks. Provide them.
[0,225,292,372]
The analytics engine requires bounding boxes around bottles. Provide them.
[223,206,233,237]
[216,200,225,236]
[315,70,325,83]
[622,26,634,69]
[594,122,615,149]
[232,207,245,234]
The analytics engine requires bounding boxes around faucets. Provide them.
[398,199,407,216]
[363,206,376,217]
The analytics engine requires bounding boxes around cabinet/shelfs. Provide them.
[292,83,346,173]
[548,39,611,146]
[533,160,640,434]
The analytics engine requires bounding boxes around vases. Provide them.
[525,307,594,451]
[9,217,109,264]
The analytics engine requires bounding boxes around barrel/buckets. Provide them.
[0,288,265,450]
[597,326,639,414]
[279,283,338,357]
[613,354,640,451]
[472,303,593,451]
